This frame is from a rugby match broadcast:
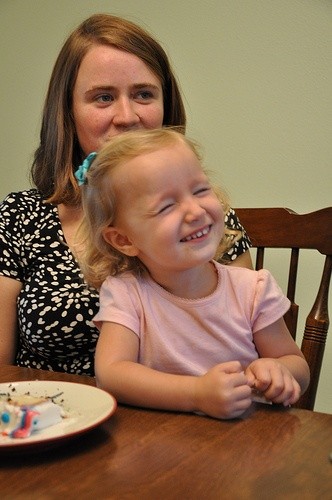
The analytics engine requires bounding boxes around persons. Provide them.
[0,14,254,376]
[78,126,310,420]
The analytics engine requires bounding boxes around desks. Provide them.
[0,362,332,500]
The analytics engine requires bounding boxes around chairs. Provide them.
[233,207,332,412]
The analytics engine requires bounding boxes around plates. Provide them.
[0,381,117,447]
